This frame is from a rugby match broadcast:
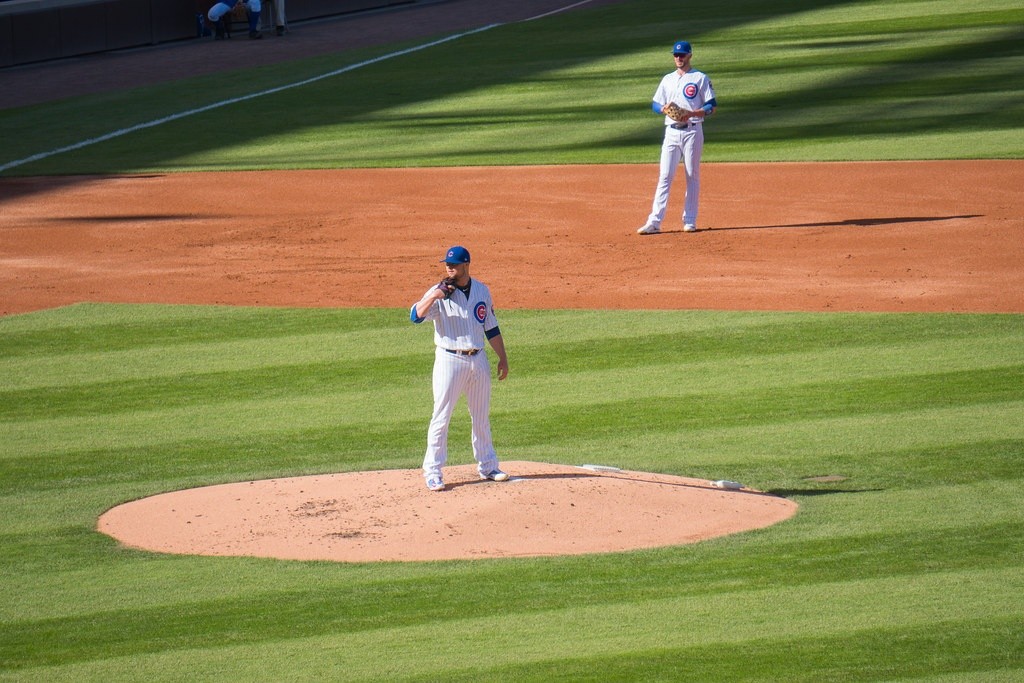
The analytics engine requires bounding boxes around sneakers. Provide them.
[637,223,660,234]
[479,468,511,482]
[684,223,697,233]
[423,470,447,490]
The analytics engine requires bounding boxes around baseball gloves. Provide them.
[664,102,684,122]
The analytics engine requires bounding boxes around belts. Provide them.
[442,348,480,356]
[671,123,697,129]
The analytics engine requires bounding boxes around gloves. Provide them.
[437,277,457,299]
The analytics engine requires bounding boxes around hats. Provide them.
[440,246,471,264]
[670,40,693,54]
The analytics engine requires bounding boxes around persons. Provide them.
[206,0,287,41]
[636,40,718,235]
[409,245,509,491]
